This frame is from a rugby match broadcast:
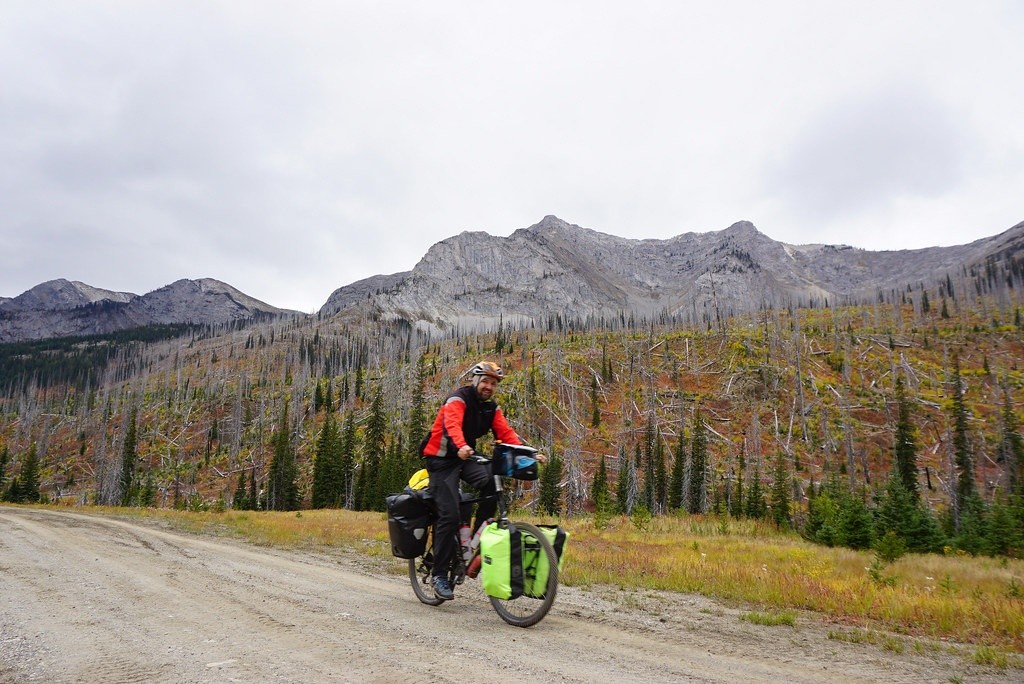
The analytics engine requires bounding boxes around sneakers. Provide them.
[429,576,454,600]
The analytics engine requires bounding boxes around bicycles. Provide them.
[407,441,559,629]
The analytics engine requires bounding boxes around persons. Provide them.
[419,362,547,600]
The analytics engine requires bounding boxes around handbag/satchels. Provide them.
[521,524,569,599]
[480,519,520,603]
[385,493,429,559]
[493,441,539,480]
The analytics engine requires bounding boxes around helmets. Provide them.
[472,361,503,382]
